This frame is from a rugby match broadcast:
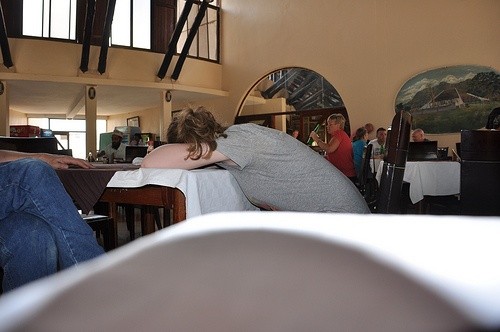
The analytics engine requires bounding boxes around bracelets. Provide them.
[317,140,322,144]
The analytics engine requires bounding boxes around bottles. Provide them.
[307,124,320,145]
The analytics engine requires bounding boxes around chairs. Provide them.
[374,110,414,214]
[407,141,439,161]
[437,146,449,161]
[356,144,374,206]
[80,214,117,252]
[125,145,149,164]
[458,130,500,216]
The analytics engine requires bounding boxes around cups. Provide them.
[102,157,108,163]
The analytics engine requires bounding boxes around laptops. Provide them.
[125,146,148,161]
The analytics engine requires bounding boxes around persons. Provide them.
[87,109,429,211]
[0,149,106,294]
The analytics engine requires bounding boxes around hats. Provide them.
[111,128,124,138]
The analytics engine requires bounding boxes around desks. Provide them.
[375,161,460,214]
[54,169,261,254]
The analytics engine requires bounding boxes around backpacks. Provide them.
[359,146,380,211]
